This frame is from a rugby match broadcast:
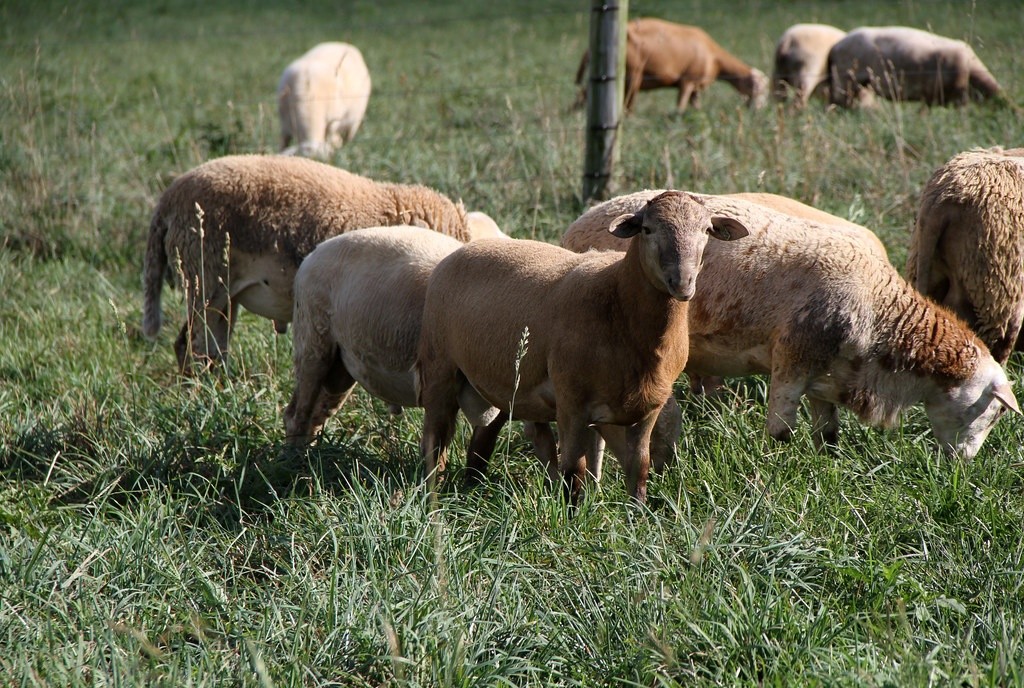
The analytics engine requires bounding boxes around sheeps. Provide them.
[276,42,371,158]
[775,24,1023,115]
[141,144,1024,514]
[565,18,769,112]
